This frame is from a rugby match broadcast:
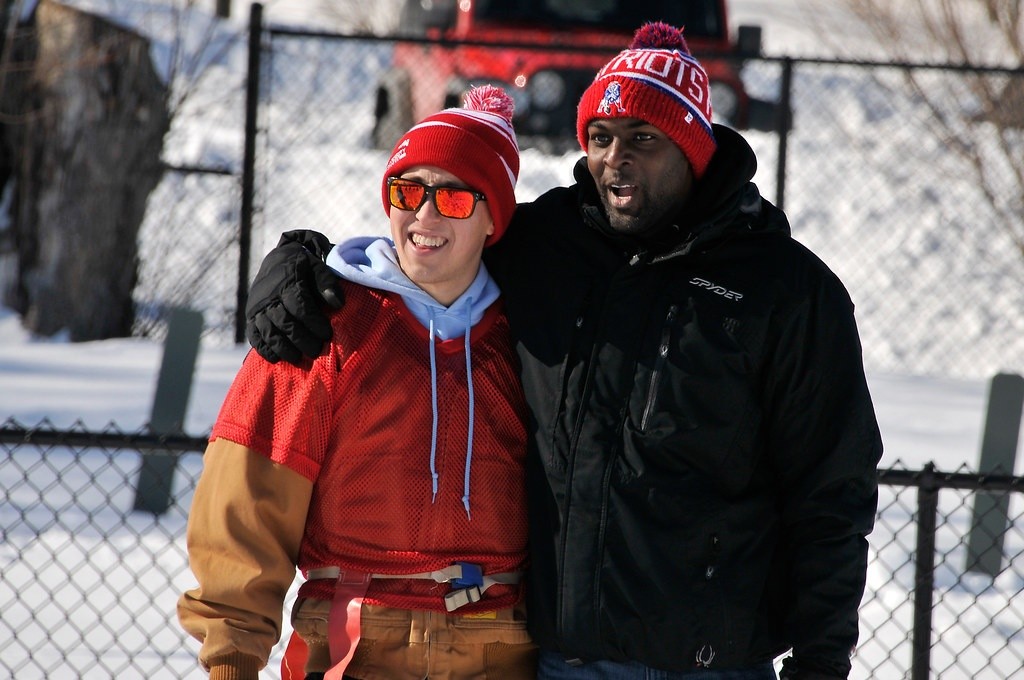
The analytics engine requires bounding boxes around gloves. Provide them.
[779,650,852,680]
[245,229,344,365]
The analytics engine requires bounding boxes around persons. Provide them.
[178,86,536,680]
[246,24,884,680]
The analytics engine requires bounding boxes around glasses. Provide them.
[387,176,487,219]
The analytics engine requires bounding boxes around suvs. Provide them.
[373,0,765,153]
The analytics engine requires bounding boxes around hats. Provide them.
[576,20,717,176]
[381,84,517,245]
